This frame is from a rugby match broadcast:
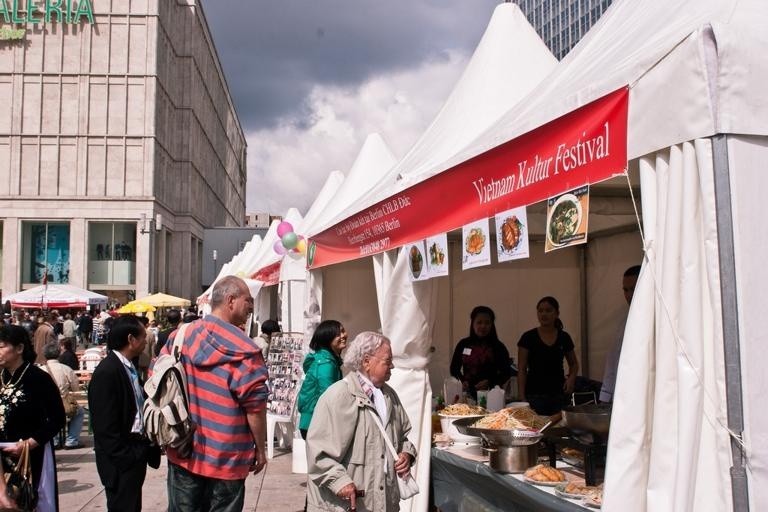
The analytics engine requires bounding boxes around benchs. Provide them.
[53,342,107,449]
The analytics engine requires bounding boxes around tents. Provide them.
[128,292,191,309]
[1,280,108,311]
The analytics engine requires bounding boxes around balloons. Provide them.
[273,222,307,260]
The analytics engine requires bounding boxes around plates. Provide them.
[546,194,582,248]
[524,475,601,507]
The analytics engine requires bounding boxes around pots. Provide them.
[479,445,529,474]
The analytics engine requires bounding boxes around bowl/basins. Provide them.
[561,405,612,437]
[437,412,495,444]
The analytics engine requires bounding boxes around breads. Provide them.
[525,463,566,483]
[564,484,590,495]
[562,446,585,457]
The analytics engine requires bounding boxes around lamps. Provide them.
[154,213,162,236]
[139,213,151,233]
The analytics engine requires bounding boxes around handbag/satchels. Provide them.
[62,394,79,415]
[396,468,419,500]
[2,439,39,512]
[143,323,196,458]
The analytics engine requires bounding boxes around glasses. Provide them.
[368,353,393,365]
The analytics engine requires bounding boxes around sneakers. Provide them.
[54,444,62,449]
[65,444,85,449]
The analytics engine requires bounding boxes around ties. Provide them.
[126,361,146,421]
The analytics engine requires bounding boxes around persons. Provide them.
[297,320,348,512]
[449,306,510,402]
[148,276,269,512]
[598,264,642,403]
[305,330,418,512]
[1,304,200,450]
[1,323,67,511]
[88,314,162,511]
[251,320,280,362]
[516,297,579,416]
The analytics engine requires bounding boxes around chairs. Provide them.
[265,384,301,460]
